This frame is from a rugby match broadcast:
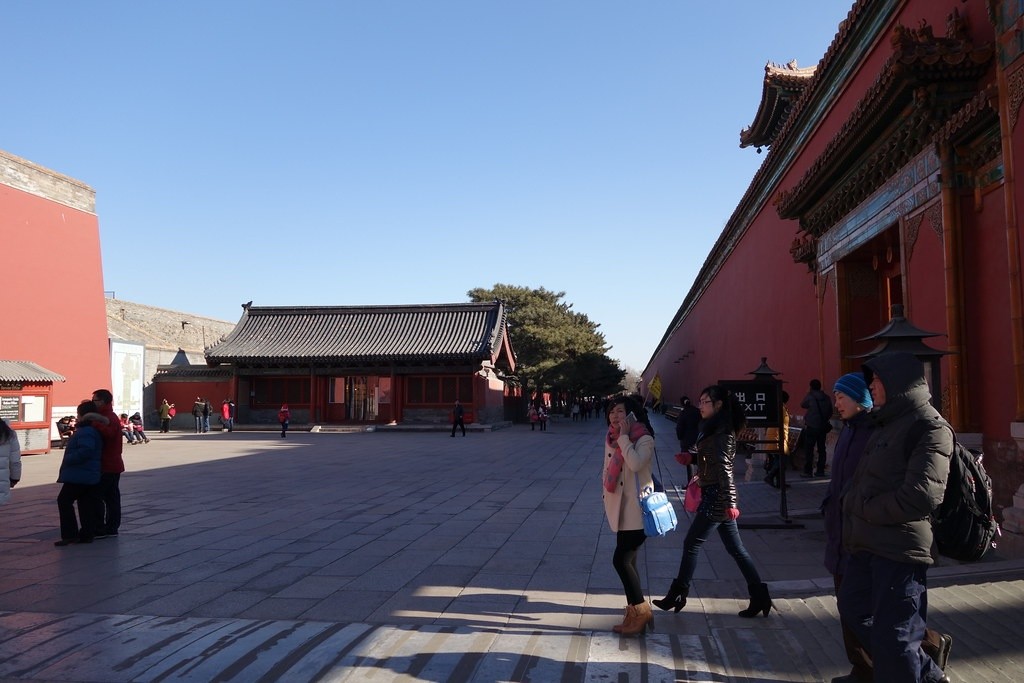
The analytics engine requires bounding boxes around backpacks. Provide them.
[904,417,1002,562]
[280,411,288,424]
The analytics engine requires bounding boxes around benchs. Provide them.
[664,405,686,424]
[56,421,73,449]
[738,426,802,473]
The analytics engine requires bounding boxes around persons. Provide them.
[158,399,171,433]
[652,396,660,414]
[602,396,656,637]
[192,396,235,433]
[677,396,704,490]
[653,385,772,618]
[526,397,614,431]
[57,416,78,449]
[449,398,465,437]
[841,351,953,683]
[129,412,149,445]
[764,390,790,488]
[0,419,21,507]
[818,373,953,683]
[120,414,139,445]
[53,400,104,546]
[797,378,833,478]
[278,403,291,440]
[82,390,125,539]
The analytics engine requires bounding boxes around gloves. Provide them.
[675,452,691,465]
[726,508,740,520]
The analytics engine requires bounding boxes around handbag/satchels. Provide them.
[683,475,701,513]
[825,422,833,433]
[531,414,539,420]
[640,491,679,538]
[651,472,666,493]
[540,414,547,421]
[169,407,176,417]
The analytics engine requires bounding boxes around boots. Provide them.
[652,578,691,612]
[738,583,771,618]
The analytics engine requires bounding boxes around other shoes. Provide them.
[132,439,151,445]
[53,534,95,546]
[764,476,775,487]
[831,666,873,683]
[800,472,813,479]
[933,633,953,671]
[94,528,118,538]
[776,482,792,488]
[937,673,949,683]
[815,471,826,477]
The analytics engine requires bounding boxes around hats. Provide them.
[833,372,874,409]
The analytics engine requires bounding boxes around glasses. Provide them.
[872,376,882,386]
[698,400,712,408]
[91,399,102,402]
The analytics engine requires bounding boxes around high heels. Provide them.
[613,601,655,637]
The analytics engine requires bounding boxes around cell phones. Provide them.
[622,411,638,425]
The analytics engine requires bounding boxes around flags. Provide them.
[647,370,662,409]
